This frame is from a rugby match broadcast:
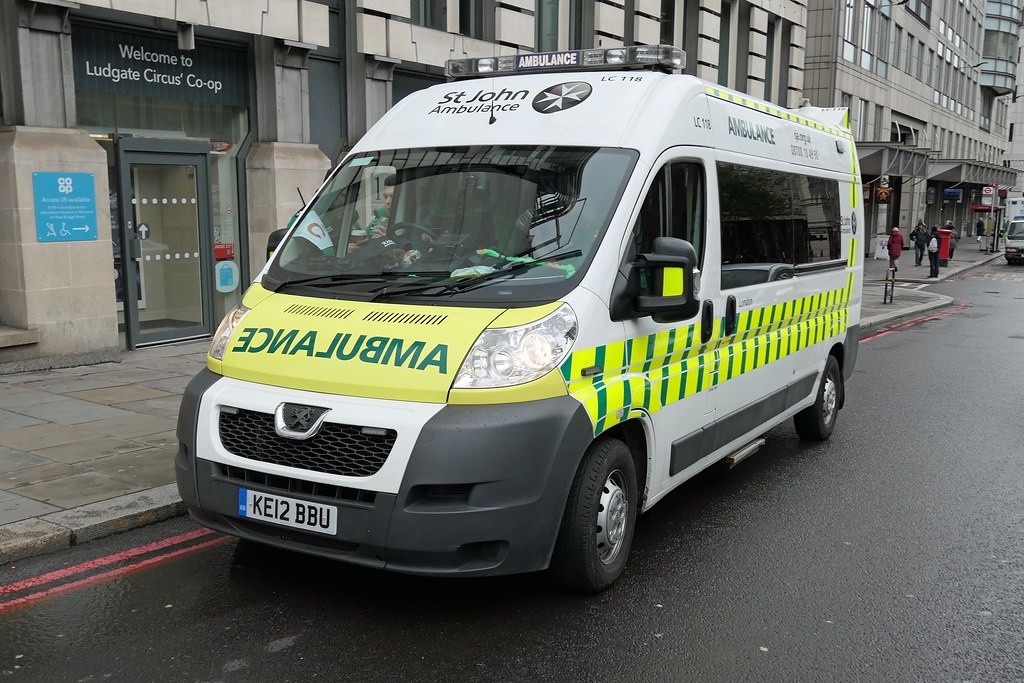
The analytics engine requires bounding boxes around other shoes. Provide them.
[895,266,897,271]
[915,263,922,266]
[926,275,939,278]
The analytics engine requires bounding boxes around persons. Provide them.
[976,216,985,243]
[1002,218,1010,243]
[501,162,644,269]
[886,218,959,278]
[359,172,396,241]
[286,167,359,258]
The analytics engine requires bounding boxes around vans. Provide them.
[1004,215,1024,266]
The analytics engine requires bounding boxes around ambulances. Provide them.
[175,44,864,593]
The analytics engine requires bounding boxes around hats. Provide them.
[918,219,924,226]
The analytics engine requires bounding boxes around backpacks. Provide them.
[928,238,938,253]
